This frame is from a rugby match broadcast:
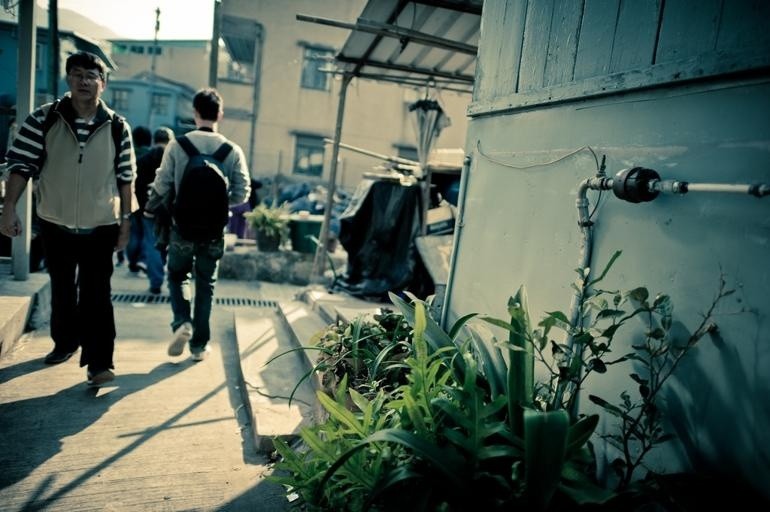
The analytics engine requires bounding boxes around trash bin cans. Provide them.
[290,210,325,254]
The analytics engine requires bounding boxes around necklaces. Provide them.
[74,99,95,117]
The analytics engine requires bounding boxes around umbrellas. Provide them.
[409,76,451,178]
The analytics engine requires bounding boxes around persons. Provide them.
[0,52,136,388]
[145,89,252,362]
[7,106,175,293]
[228,180,262,239]
[308,184,328,215]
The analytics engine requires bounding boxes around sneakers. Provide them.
[87,366,115,387]
[191,344,212,362]
[44,346,79,363]
[168,322,194,356]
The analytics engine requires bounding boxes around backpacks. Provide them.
[175,133,235,235]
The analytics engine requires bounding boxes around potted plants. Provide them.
[242,195,298,253]
